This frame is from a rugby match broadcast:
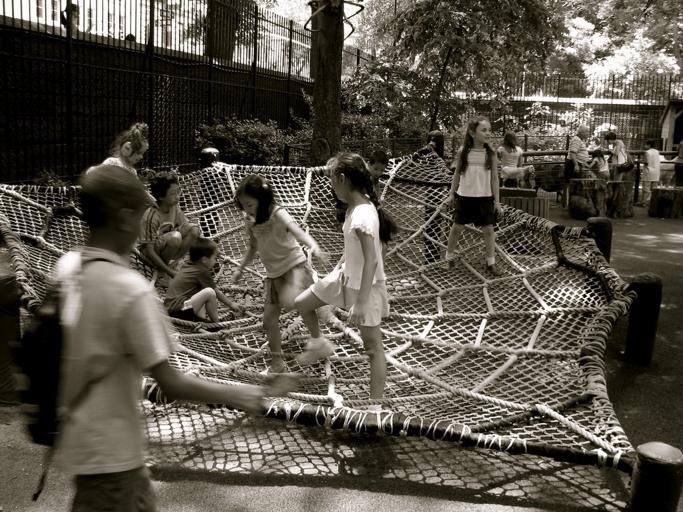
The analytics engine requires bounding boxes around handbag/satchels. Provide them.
[615,162,634,174]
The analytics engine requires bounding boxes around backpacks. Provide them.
[20,258,122,447]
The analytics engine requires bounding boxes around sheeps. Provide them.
[499,164,536,188]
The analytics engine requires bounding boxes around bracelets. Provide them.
[238,269,243,274]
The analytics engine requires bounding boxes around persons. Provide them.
[495,124,683,206]
[294,150,390,401]
[85,122,244,324]
[43,165,264,512]
[231,174,329,380]
[444,116,503,275]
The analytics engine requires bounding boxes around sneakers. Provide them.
[297,339,333,365]
[446,257,456,270]
[259,365,284,376]
[486,261,501,276]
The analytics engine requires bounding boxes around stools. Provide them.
[566,178,637,220]
[647,186,681,219]
